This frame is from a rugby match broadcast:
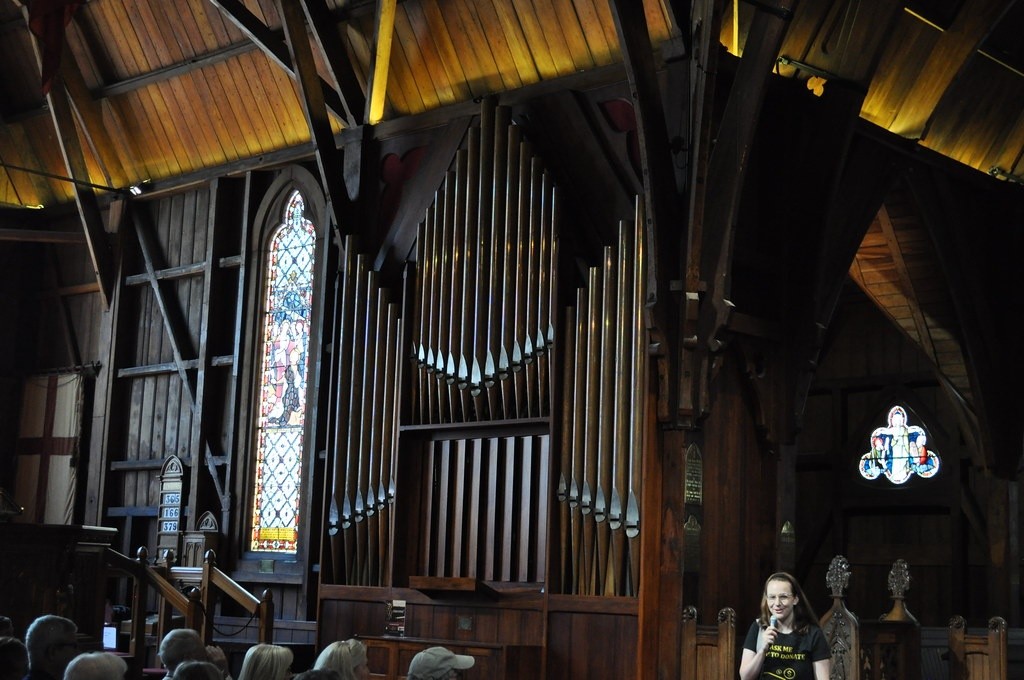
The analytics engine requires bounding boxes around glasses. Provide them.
[339,640,351,655]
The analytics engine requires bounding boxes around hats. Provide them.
[409,647,475,679]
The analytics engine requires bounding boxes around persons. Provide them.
[0,616,127,680]
[159,630,370,680]
[739,571,833,680]
[406,646,475,680]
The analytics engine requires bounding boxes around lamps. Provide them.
[128,181,146,196]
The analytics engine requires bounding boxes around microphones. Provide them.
[771,614,778,628]
